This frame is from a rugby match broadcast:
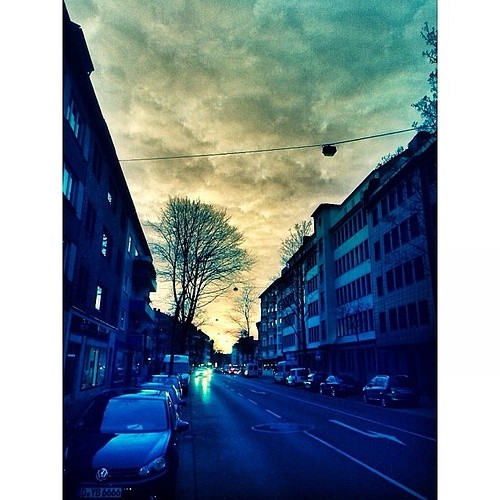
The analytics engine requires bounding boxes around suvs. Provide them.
[286,367,311,387]
[361,374,419,409]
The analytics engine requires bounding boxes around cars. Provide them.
[63,382,192,500]
[303,373,327,392]
[214,363,262,378]
[132,373,186,416]
[318,374,360,399]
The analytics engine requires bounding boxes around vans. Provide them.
[273,361,300,385]
[160,354,191,386]
